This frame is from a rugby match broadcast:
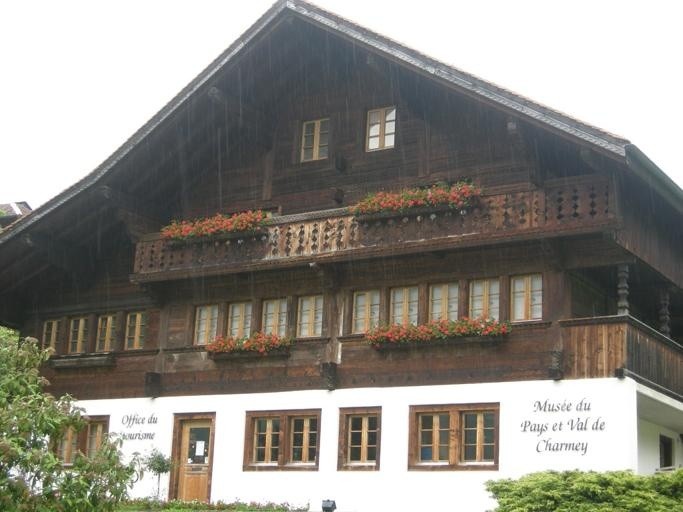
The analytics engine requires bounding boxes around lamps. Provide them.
[321,498,336,512]
